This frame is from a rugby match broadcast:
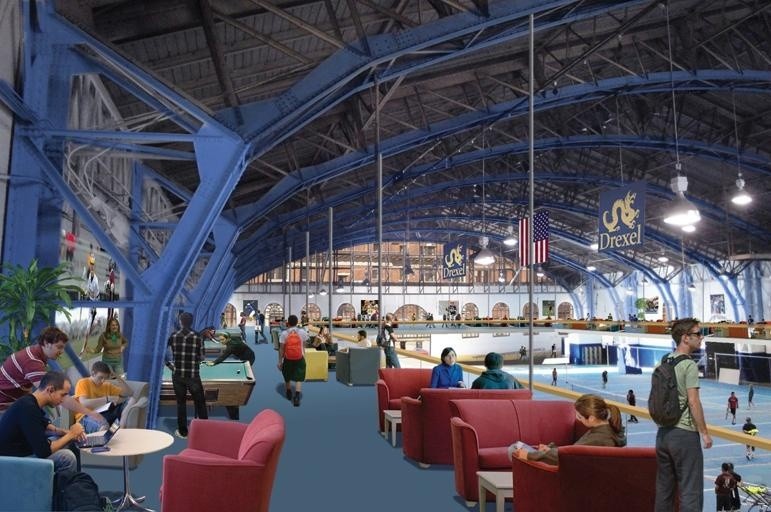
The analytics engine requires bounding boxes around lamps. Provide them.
[502,157,520,247]
[660,0,702,227]
[726,82,756,208]
[473,129,496,268]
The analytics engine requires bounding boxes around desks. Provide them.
[73,422,174,512]
[155,359,256,421]
[203,327,244,357]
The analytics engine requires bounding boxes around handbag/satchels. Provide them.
[57,473,106,512]
[376,325,392,347]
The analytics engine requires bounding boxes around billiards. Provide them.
[236,369,240,374]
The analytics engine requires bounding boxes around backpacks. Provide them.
[647,353,692,428]
[285,328,302,360]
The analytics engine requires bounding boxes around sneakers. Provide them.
[175,429,188,440]
[293,397,299,406]
[286,392,292,400]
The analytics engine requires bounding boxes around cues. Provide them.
[201,361,245,364]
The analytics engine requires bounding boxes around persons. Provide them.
[0,371,82,488]
[588,312,638,321]
[73,361,134,440]
[238,310,268,344]
[84,317,129,379]
[207,332,255,366]
[0,327,106,444]
[381,313,401,368]
[714,462,736,512]
[507,393,627,465]
[429,347,466,389]
[200,327,220,345]
[282,310,370,352]
[65,227,116,302]
[277,314,306,407]
[655,318,713,512]
[727,463,742,512]
[471,352,525,390]
[519,345,758,460]
[426,313,461,328]
[165,312,208,439]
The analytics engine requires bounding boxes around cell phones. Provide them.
[92,446,111,453]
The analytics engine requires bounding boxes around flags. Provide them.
[517,210,550,266]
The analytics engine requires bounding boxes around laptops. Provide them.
[80,421,121,448]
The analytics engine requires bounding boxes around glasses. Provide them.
[679,330,701,336]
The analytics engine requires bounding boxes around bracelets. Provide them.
[106,396,109,404]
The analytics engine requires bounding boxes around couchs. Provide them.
[399,385,532,470]
[160,409,287,512]
[302,346,330,383]
[269,319,338,356]
[510,442,657,511]
[374,366,434,433]
[335,346,382,386]
[1,429,60,511]
[449,397,589,510]
[60,377,151,471]
